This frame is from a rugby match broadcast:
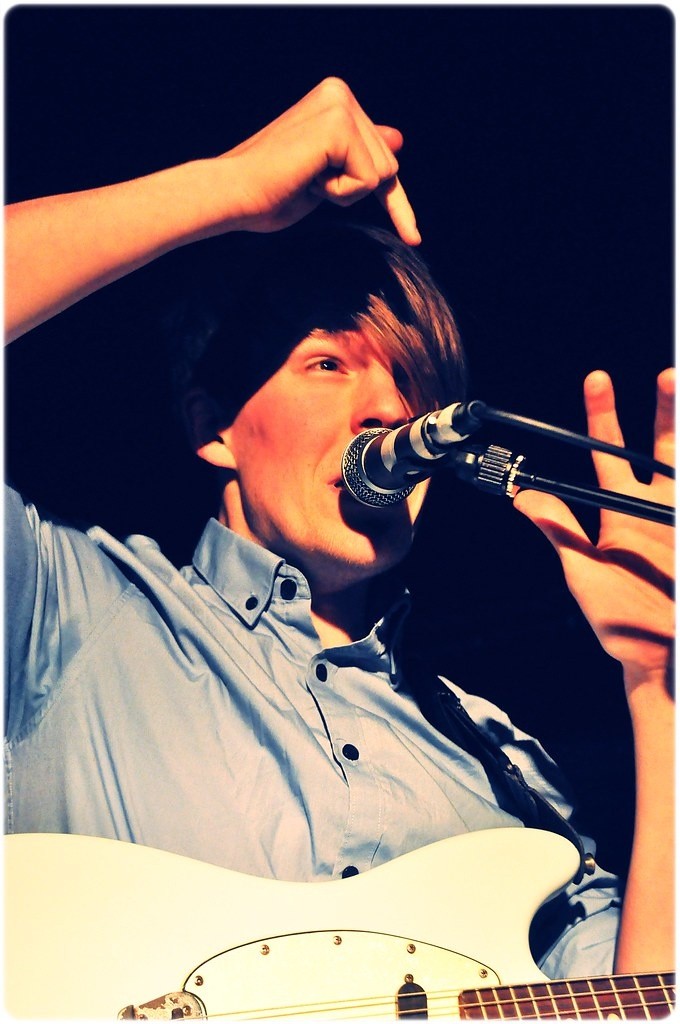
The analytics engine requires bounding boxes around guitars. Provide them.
[2,825,679,1022]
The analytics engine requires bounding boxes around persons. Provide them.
[3,76,676,980]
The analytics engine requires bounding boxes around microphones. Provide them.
[341,401,487,507]
